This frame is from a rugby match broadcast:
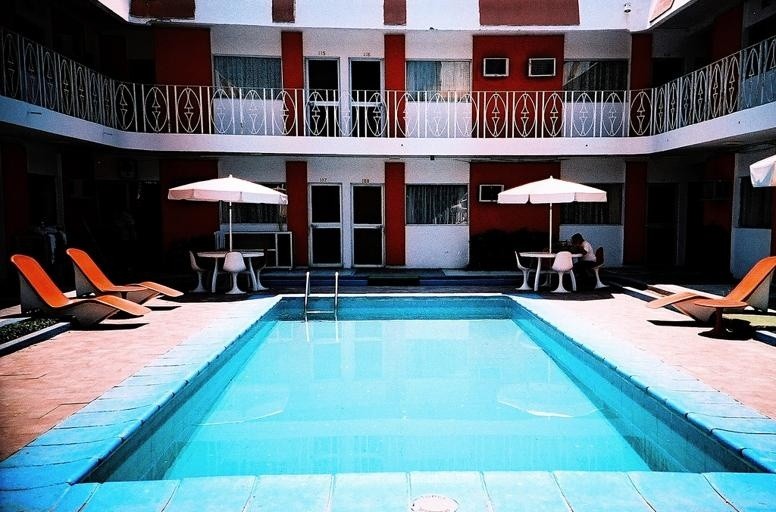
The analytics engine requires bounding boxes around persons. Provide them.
[571,234,597,292]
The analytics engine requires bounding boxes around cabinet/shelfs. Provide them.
[224,231,295,270]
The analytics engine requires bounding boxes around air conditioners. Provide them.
[478,183,505,204]
[483,57,510,77]
[528,57,556,77]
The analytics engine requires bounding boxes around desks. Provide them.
[694,300,748,333]
[103,286,148,300]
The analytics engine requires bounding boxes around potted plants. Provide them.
[280,215,288,231]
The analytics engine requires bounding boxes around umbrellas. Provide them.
[167,174,289,290]
[749,156,776,188]
[498,175,608,284]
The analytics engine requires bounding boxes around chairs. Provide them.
[513,245,609,294]
[10,253,151,326]
[65,247,183,305]
[646,255,776,332]
[187,246,271,295]
